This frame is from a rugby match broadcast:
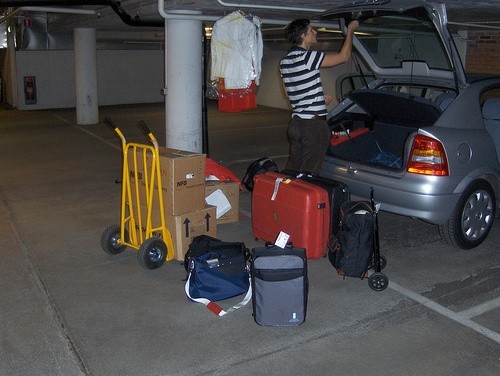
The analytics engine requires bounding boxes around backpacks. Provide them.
[242,154,280,192]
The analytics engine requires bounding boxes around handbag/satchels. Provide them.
[327,122,381,161]
[183,234,251,315]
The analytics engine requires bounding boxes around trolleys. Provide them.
[100,116,175,271]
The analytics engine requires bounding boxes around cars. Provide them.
[313,0,500,251]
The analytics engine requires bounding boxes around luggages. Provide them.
[249,242,309,329]
[330,186,389,291]
[249,167,351,260]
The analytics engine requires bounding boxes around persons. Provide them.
[278,18,359,172]
